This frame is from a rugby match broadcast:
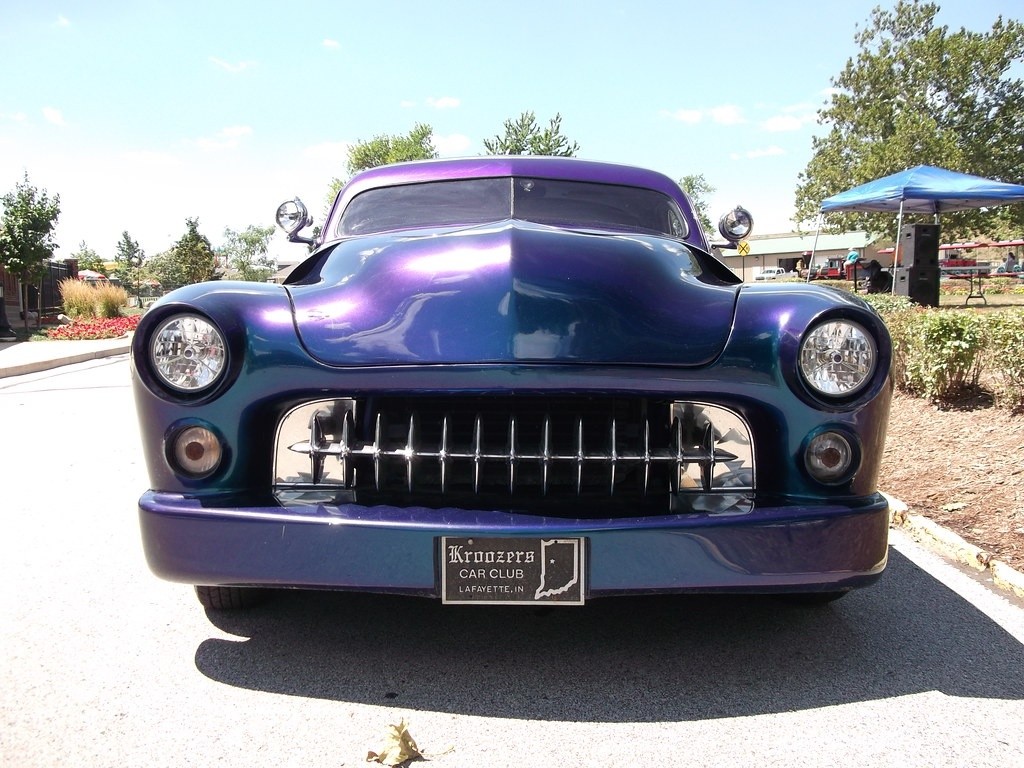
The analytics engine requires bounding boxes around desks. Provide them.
[880,266,997,305]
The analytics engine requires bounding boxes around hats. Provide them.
[848,247,856,250]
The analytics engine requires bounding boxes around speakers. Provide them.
[895,267,940,308]
[902,224,940,266]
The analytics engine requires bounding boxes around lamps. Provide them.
[755,256,759,261]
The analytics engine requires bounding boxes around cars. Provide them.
[124,154,896,611]
[78,269,106,285]
[755,267,785,280]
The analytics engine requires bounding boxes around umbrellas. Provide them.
[78,269,105,280]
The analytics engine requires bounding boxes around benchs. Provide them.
[855,257,867,262]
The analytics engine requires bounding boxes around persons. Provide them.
[796,259,803,276]
[1001,258,1007,268]
[841,247,859,273]
[1005,252,1015,272]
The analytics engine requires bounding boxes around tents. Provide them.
[806,164,1024,295]
